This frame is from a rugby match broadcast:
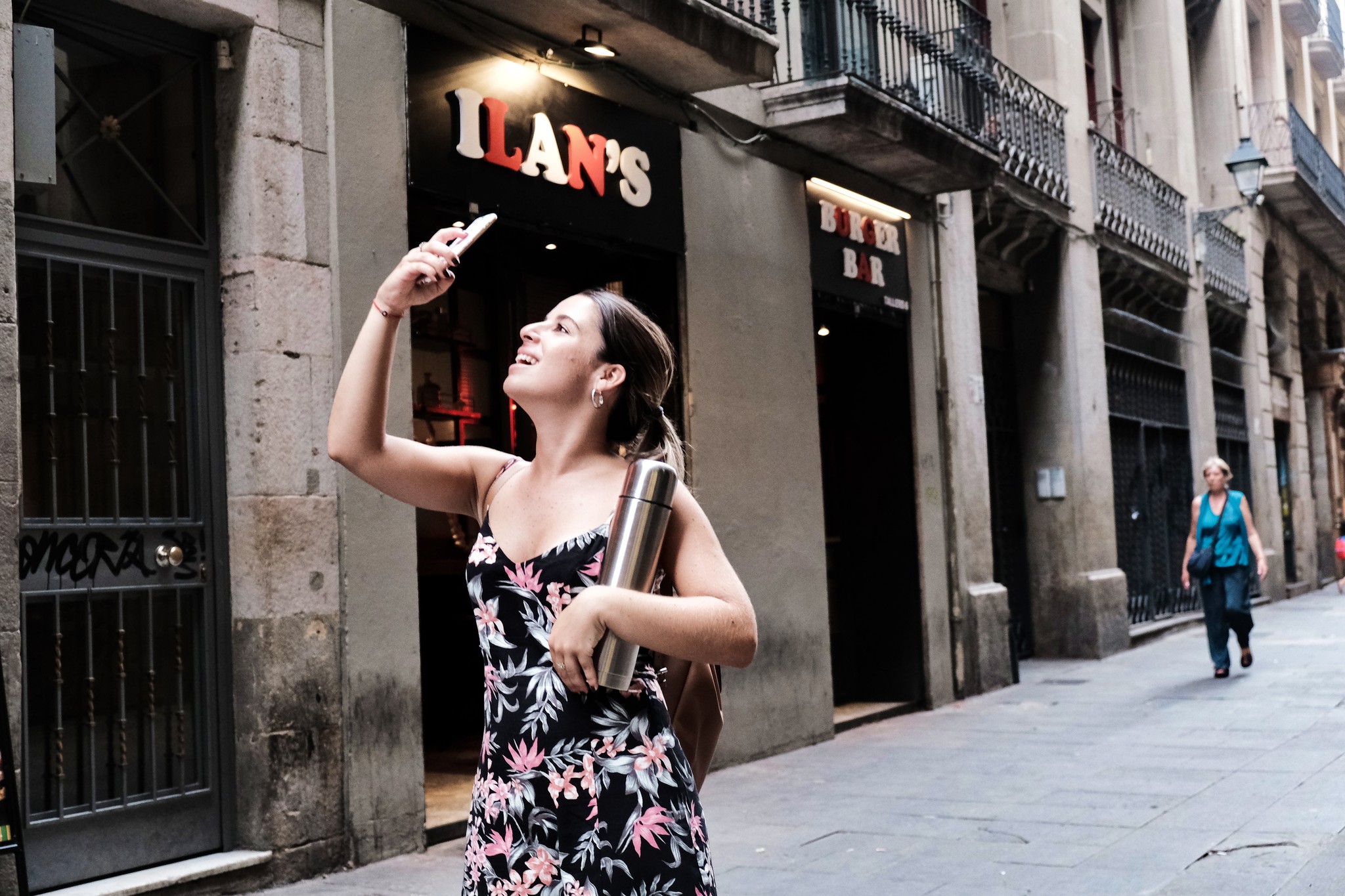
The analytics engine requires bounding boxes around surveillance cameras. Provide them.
[215,39,230,70]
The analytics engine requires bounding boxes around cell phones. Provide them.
[417,213,498,285]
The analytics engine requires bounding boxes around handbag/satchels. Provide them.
[1335,537,1345,560]
[1187,539,1216,578]
[649,593,728,793]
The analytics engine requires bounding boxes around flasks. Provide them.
[584,458,679,693]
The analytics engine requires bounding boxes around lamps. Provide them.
[570,21,621,64]
[1195,133,1272,232]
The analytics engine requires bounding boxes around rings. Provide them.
[418,242,428,252]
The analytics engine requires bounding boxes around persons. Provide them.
[1180,455,1267,678]
[1335,492,1345,595]
[326,229,759,896]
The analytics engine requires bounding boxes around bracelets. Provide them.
[371,300,404,320]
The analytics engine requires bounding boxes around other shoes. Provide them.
[1241,652,1253,667]
[1214,668,1230,678]
